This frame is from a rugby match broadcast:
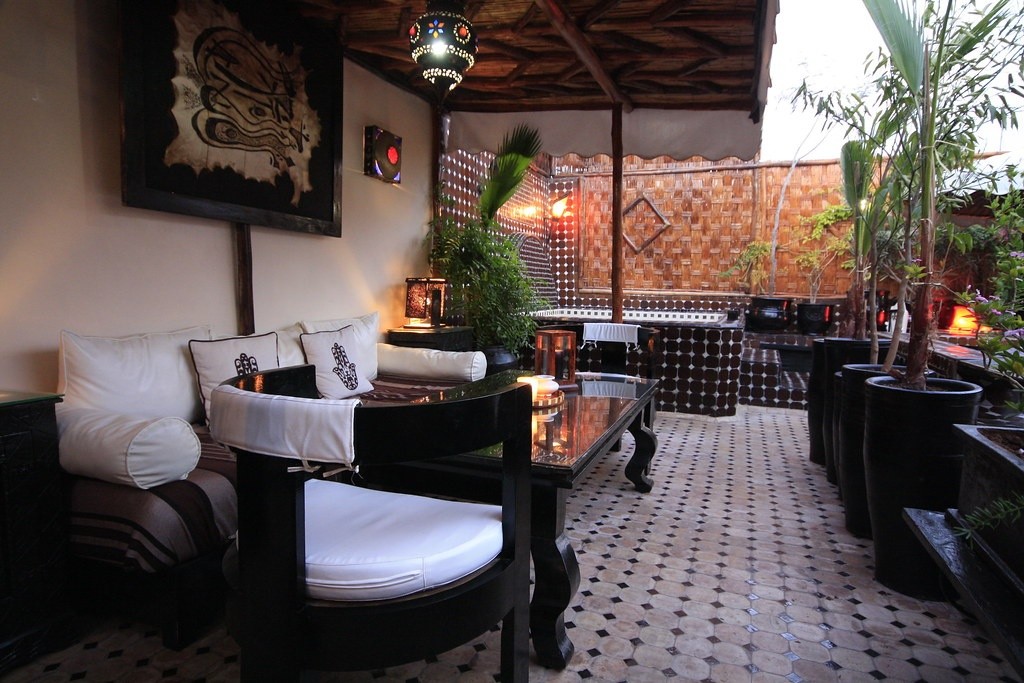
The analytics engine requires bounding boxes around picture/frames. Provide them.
[119,0,345,238]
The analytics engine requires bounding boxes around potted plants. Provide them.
[861,0,1024,594]
[807,118,898,507]
[423,122,545,369]
[844,0,1018,539]
[718,241,795,333]
[947,177,1024,605]
[789,189,856,336]
[854,229,903,330]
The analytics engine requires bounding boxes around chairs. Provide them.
[534,323,661,477]
[209,366,533,683]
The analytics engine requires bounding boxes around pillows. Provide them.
[54,326,213,424]
[299,325,374,402]
[268,322,308,367]
[377,342,488,382]
[298,311,382,345]
[189,331,281,431]
[54,400,202,489]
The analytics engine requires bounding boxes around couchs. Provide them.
[61,376,475,652]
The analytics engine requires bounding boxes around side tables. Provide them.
[387,324,476,352]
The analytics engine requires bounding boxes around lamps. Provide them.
[404,278,447,330]
[544,192,571,247]
[361,125,401,184]
[407,0,481,87]
[533,330,579,394]
[518,376,537,396]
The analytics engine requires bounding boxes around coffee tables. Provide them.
[364,368,660,673]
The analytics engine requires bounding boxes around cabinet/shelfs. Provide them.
[0,388,86,678]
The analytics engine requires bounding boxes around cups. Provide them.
[517,377,538,401]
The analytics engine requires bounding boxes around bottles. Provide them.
[534,374,560,396]
